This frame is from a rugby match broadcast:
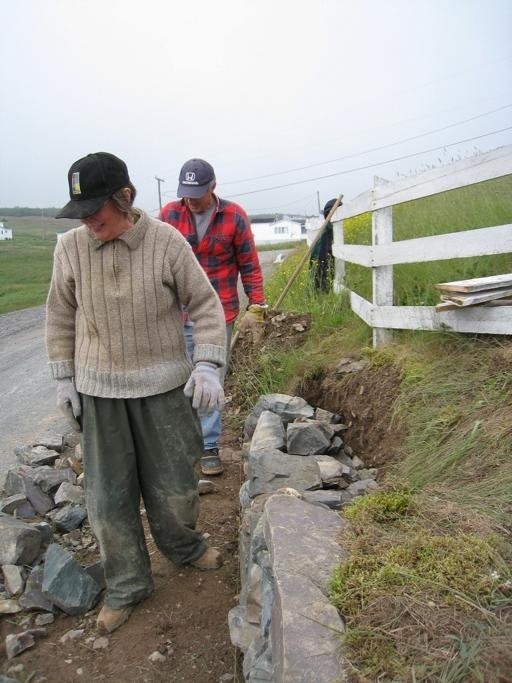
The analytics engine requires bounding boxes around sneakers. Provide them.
[95,605,130,635]
[199,448,223,475]
[189,547,222,571]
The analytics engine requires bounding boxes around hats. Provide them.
[177,160,214,198]
[55,153,128,219]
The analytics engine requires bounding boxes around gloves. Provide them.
[55,378,83,432]
[185,361,224,410]
[236,304,267,348]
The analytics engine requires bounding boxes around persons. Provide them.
[156,157,268,477]
[43,151,229,635]
